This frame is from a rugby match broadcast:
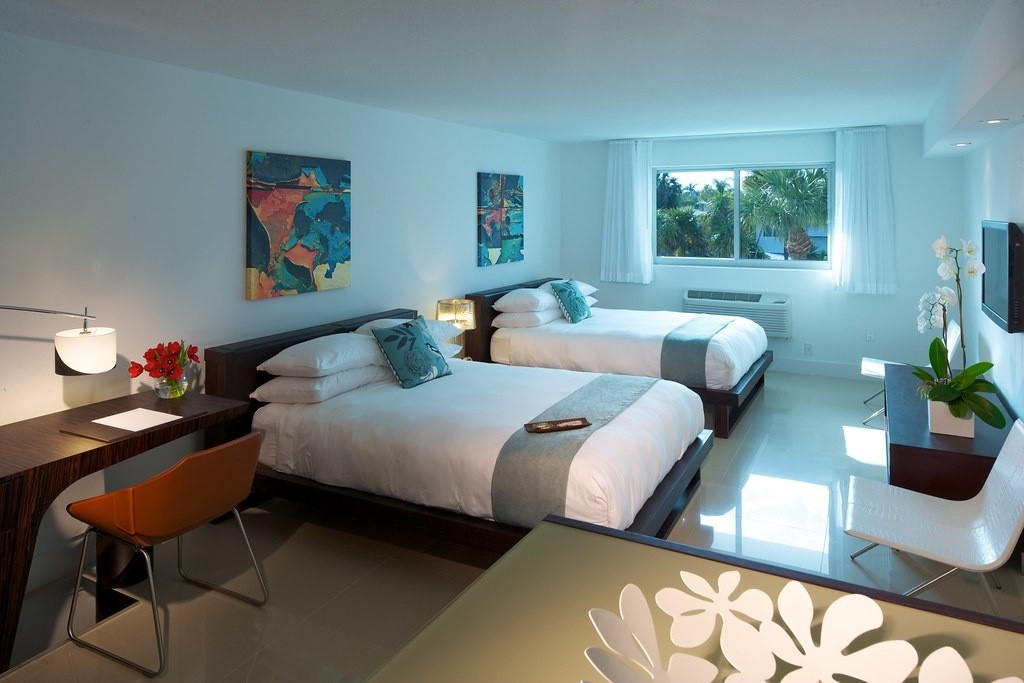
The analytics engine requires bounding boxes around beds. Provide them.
[463,277,773,439]
[203,308,716,563]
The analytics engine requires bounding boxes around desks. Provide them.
[1,388,251,673]
[354,514,1024,683]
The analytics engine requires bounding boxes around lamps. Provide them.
[435,298,476,358]
[0,304,117,376]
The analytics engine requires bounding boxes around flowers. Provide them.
[899,233,1005,430]
[129,339,202,397]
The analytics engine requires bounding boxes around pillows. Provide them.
[370,314,453,391]
[253,333,392,376]
[354,316,465,342]
[247,366,392,402]
[538,280,600,296]
[490,287,558,312]
[550,277,593,325]
[490,309,563,328]
[436,342,464,362]
[586,296,597,308]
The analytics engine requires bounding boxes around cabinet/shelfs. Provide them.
[881,363,1017,501]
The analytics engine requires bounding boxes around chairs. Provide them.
[861,318,960,425]
[67,431,269,679]
[843,417,1024,597]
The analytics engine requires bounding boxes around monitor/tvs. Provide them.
[981,219,1024,334]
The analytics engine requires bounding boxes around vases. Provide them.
[925,393,975,438]
[153,370,189,399]
